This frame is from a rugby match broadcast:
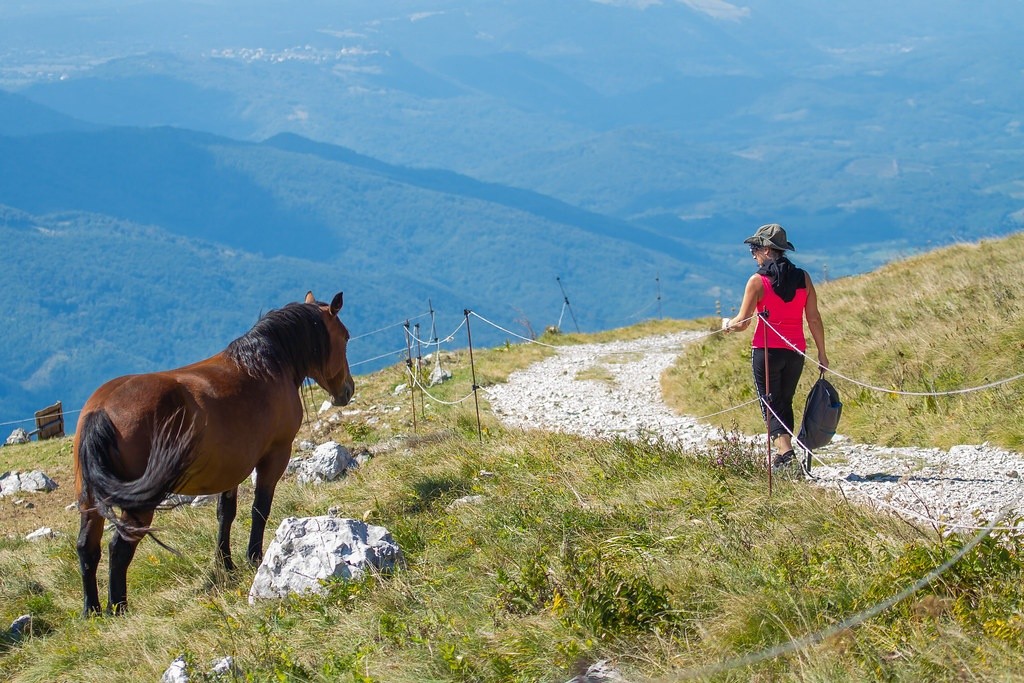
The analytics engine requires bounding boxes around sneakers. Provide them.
[771,449,796,473]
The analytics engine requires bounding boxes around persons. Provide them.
[720,224,828,477]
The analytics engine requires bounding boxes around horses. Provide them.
[74,291,354,619]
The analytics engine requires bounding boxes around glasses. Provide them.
[749,248,758,255]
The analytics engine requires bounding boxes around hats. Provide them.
[743,224,795,252]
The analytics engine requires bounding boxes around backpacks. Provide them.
[797,372,842,471]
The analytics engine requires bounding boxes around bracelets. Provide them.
[726,320,730,331]
